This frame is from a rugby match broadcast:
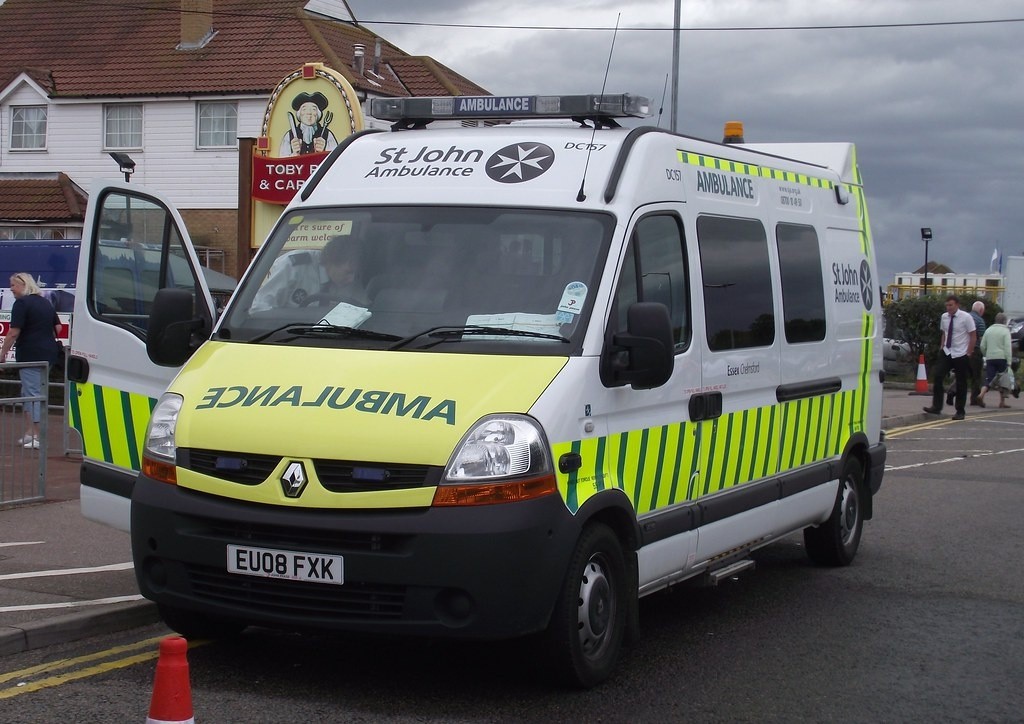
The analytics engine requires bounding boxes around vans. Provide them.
[0,238,240,407]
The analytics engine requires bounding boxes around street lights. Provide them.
[920,227,933,295]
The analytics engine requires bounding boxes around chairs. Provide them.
[358,240,451,313]
[543,254,595,316]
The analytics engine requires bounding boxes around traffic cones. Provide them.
[146,634,195,724]
[908,354,936,396]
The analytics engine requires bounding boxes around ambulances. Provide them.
[67,93,888,695]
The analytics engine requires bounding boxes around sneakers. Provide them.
[17,432,38,447]
[23,438,39,450]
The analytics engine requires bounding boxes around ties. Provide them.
[946,315,955,348]
[320,280,332,307]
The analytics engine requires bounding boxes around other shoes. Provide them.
[946,392,953,406]
[998,402,1011,408]
[976,395,985,408]
[923,405,940,414]
[970,401,978,406]
[952,412,964,420]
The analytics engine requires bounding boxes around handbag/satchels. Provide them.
[57,339,66,371]
[988,366,1015,394]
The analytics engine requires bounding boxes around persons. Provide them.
[250,234,363,309]
[923,296,977,419]
[0,272,62,449]
[976,312,1012,408]
[946,301,985,406]
